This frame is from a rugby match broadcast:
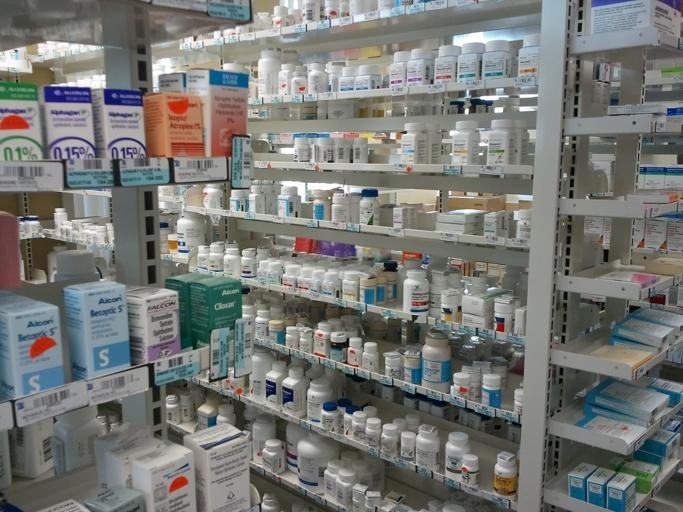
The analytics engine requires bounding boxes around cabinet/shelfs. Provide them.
[545,0,683,512]
[2,2,257,512]
[172,2,543,512]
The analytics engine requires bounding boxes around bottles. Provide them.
[481,373,502,409]
[517,34,540,76]
[286,422,309,474]
[248,347,274,401]
[262,185,276,214]
[196,245,210,269]
[343,405,360,437]
[388,51,411,85]
[514,119,529,166]
[452,372,471,401]
[36,41,103,61]
[352,137,369,163]
[493,451,518,501]
[293,137,310,163]
[380,424,399,458]
[341,306,352,316]
[328,318,342,332]
[400,431,416,463]
[216,403,237,426]
[256,245,400,305]
[281,367,310,418]
[229,189,246,213]
[433,45,461,82]
[208,243,224,272]
[341,315,358,338]
[405,414,420,434]
[364,417,382,449]
[312,190,331,220]
[393,419,406,454]
[242,404,258,431]
[288,356,306,371]
[313,322,332,358]
[201,184,223,209]
[176,211,205,257]
[241,289,285,318]
[421,331,452,393]
[180,393,194,422]
[334,138,351,163]
[364,500,378,512]
[166,394,181,425]
[445,432,471,483]
[239,249,257,282]
[331,197,349,223]
[493,302,512,334]
[427,124,442,164]
[430,274,448,319]
[451,120,480,165]
[352,411,367,443]
[48,74,107,88]
[336,398,352,430]
[223,247,241,276]
[261,493,280,512]
[461,453,479,489]
[306,380,334,427]
[53,205,114,246]
[323,459,344,501]
[349,195,360,224]
[352,491,364,512]
[404,357,421,386]
[254,309,269,339]
[402,269,430,316]
[400,122,428,164]
[385,357,401,379]
[486,119,516,165]
[184,185,203,207]
[257,47,381,96]
[52,249,100,284]
[299,331,313,354]
[269,320,285,346]
[249,185,265,214]
[347,337,364,368]
[277,186,301,218]
[352,460,373,490]
[481,40,513,78]
[516,208,531,239]
[341,450,358,469]
[265,361,289,405]
[297,431,340,494]
[456,43,485,82]
[364,453,385,492]
[285,326,299,350]
[439,293,459,323]
[317,137,333,162]
[361,342,379,372]
[415,424,440,471]
[287,294,323,326]
[46,245,68,283]
[261,438,286,475]
[230,371,245,395]
[50,403,103,475]
[197,398,220,430]
[359,189,380,226]
[329,331,347,363]
[17,215,41,239]
[252,414,276,464]
[319,366,347,399]
[211,0,428,36]
[337,468,360,511]
[514,388,523,415]
[304,363,324,381]
[406,48,434,85]
[324,303,339,319]
[243,430,253,461]
[364,406,378,419]
[321,402,339,433]
